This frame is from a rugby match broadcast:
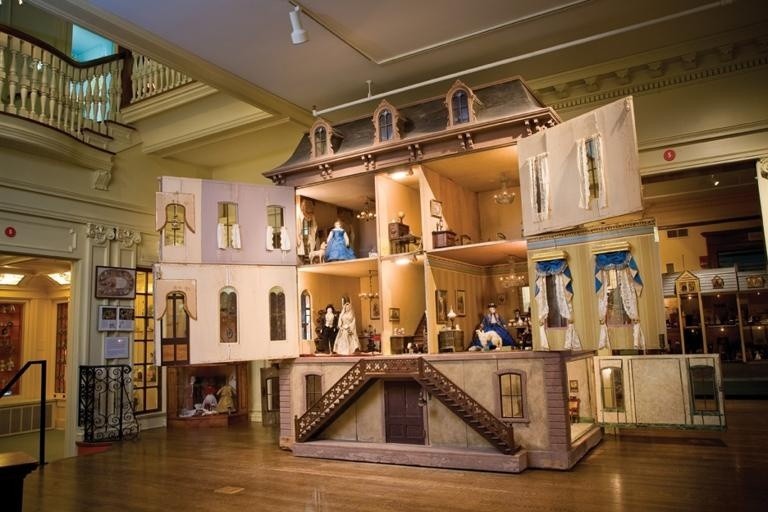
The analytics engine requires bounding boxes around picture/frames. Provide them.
[431,199,443,219]
[436,289,466,324]
[95,265,137,301]
[370,295,380,320]
[389,308,400,321]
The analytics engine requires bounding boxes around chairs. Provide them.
[569,396,580,423]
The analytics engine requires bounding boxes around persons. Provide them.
[333,302,362,356]
[201,384,219,416]
[216,383,238,413]
[325,220,357,261]
[318,305,337,339]
[468,302,515,350]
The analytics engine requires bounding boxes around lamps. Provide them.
[494,173,515,205]
[498,255,525,288]
[358,270,379,299]
[356,196,376,223]
[288,6,310,45]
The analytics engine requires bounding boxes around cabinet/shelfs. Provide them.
[391,338,414,353]
[666,290,768,363]
[440,332,464,353]
[0,403,54,437]
[389,223,409,253]
[262,76,606,474]
[434,233,455,248]
